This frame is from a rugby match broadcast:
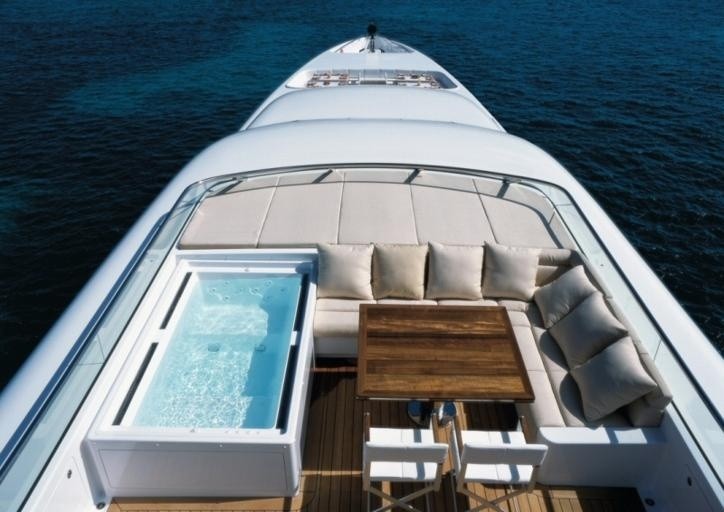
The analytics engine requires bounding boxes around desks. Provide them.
[357,301,535,423]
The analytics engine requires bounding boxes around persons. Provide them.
[367,21,376,39]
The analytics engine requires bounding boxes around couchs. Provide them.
[312,241,670,489]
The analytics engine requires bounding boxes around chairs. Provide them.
[358,424,548,512]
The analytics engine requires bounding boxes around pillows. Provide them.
[549,290,626,370]
[425,239,483,299]
[536,263,596,329]
[370,240,429,302]
[314,242,376,301]
[571,336,657,423]
[482,240,541,303]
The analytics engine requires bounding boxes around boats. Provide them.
[0,15,722,511]
